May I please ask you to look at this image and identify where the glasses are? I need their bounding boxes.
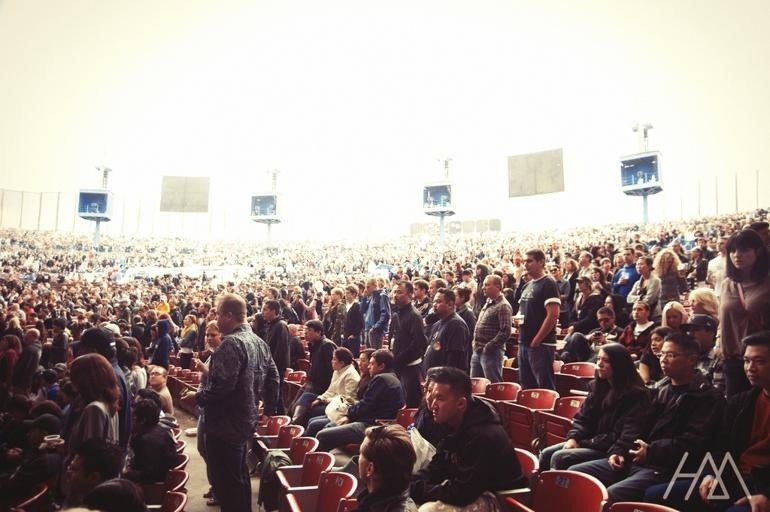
[655,350,696,360]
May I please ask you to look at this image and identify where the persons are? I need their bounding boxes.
[299,288,318,319]
[645,332,770,511]
[688,315,728,392]
[287,318,340,417]
[588,268,611,295]
[306,349,408,449]
[518,207,770,252]
[564,276,603,341]
[611,247,638,296]
[341,285,363,360]
[563,259,579,282]
[410,368,530,512]
[180,291,280,511]
[578,251,598,278]
[322,288,346,346]
[422,287,469,376]
[618,300,658,350]
[539,343,651,487]
[567,334,728,504]
[298,234,516,286]
[687,247,707,288]
[626,257,663,326]
[599,258,612,282]
[195,320,223,508]
[180,237,297,317]
[414,369,454,445]
[469,274,514,383]
[587,305,623,346]
[651,248,689,310]
[352,424,420,512]
[261,300,305,416]
[661,301,689,332]
[706,237,730,287]
[180,314,196,369]
[385,282,429,408]
[294,348,362,429]
[308,348,379,422]
[362,279,389,349]
[517,247,562,392]
[713,230,770,394]
[688,286,718,320]
[639,326,674,386]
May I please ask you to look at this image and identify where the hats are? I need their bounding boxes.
[678,314,718,332]
[66,327,115,348]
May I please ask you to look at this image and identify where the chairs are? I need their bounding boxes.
[474,382,521,412]
[496,470,609,512]
[554,362,596,396]
[257,425,304,461]
[499,388,561,450]
[258,400,263,407]
[10,482,49,512]
[147,492,187,512]
[501,365,520,383]
[375,408,420,429]
[258,407,264,416]
[253,416,291,438]
[283,471,358,512]
[266,436,320,464]
[276,452,335,489]
[535,395,588,447]
[168,470,189,491]
[470,377,491,395]
[513,447,540,486]
[175,440,186,455]
[173,452,190,470]
[282,367,307,407]
[167,364,200,392]
[609,501,679,512]
[174,428,182,439]
